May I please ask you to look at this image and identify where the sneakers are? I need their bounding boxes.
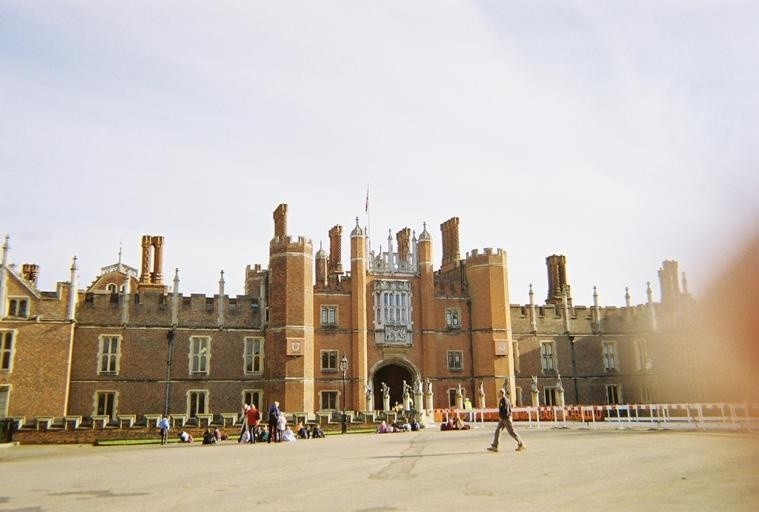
[515,444,525,451]
[487,447,498,452]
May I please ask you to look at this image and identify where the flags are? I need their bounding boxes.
[365,191,369,213]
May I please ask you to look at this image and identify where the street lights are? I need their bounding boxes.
[163,329,176,417]
[567,335,581,405]
[338,351,350,436]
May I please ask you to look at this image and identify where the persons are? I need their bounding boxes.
[244,403,260,445]
[341,411,347,434]
[176,426,229,444]
[487,388,526,452]
[463,397,473,409]
[158,413,171,445]
[374,412,465,434]
[236,402,251,444]
[268,400,281,443]
[276,410,287,442]
[253,419,325,442]
